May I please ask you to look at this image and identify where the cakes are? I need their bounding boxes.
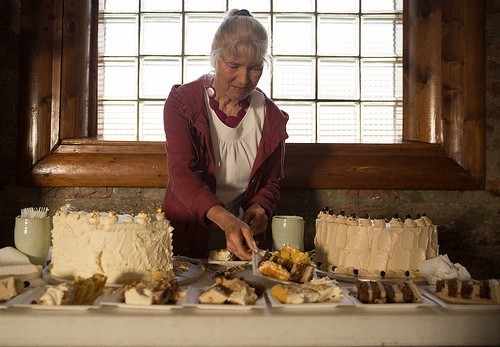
[270,275,342,304]
[357,281,422,303]
[433,278,500,303]
[39,273,108,305]
[313,207,439,279]
[51,203,173,285]
[121,278,179,306]
[199,277,257,306]
[258,245,313,283]
[0,274,25,300]
[208,248,239,261]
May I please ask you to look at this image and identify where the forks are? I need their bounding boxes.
[20,207,48,218]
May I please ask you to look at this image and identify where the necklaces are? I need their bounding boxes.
[206,85,249,128]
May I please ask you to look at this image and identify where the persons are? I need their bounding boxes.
[160,10,289,261]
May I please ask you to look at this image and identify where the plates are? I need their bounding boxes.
[207,255,253,268]
[182,286,268,311]
[420,284,500,310]
[100,281,185,310]
[344,286,437,309]
[9,284,104,312]
[266,286,355,309]
[251,248,316,285]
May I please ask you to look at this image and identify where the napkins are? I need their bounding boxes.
[0,247,38,276]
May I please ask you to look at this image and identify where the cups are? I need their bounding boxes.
[272,215,304,252]
[14,217,51,268]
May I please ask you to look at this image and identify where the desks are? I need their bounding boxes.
[0,258,500,347]
